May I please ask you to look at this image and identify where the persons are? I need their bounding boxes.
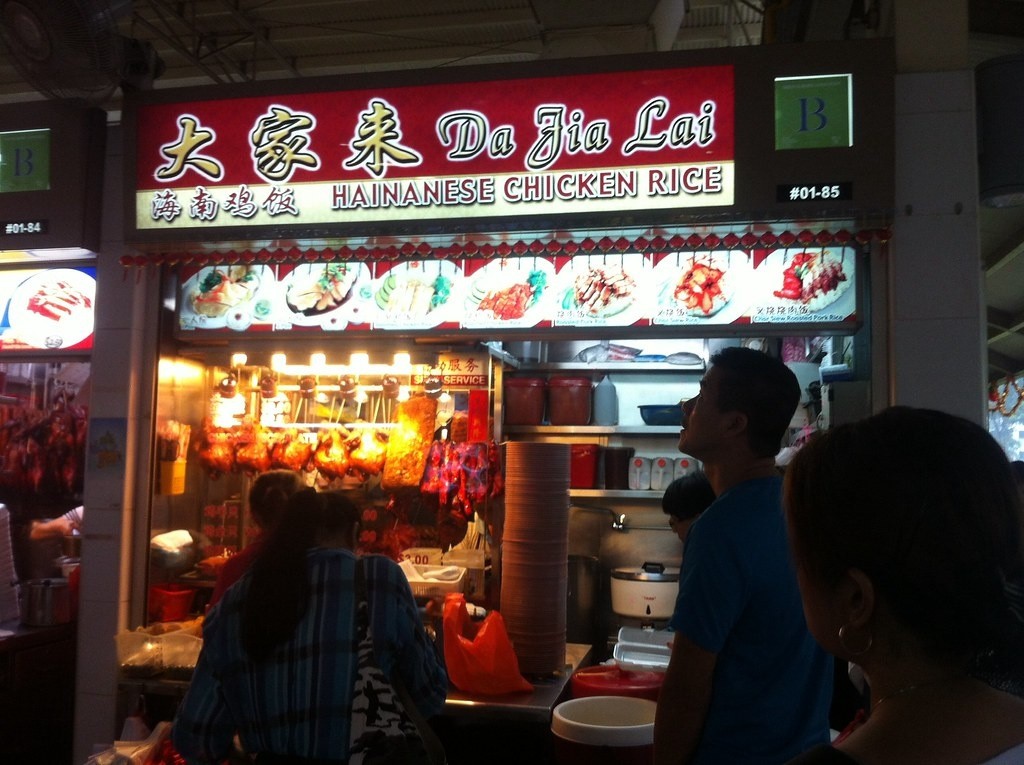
[653,348,1024,765]
[169,471,449,765]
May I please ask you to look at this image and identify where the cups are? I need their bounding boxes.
[161,461,186,495]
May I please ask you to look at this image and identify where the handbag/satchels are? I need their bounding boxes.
[425,592,534,696]
[347,555,447,765]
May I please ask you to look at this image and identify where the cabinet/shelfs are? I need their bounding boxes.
[524,357,705,501]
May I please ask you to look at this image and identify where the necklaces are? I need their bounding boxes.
[870,674,967,713]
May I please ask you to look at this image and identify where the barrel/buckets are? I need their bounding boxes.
[551,696,657,765]
[502,378,544,426]
[147,582,197,622]
[605,446,635,489]
[571,665,665,700]
[547,377,591,425]
[786,362,819,403]
[570,444,599,489]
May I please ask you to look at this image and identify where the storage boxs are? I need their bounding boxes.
[571,444,599,488]
[505,377,547,424]
[550,375,593,424]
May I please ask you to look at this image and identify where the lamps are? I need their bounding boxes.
[299,376,319,401]
[257,374,278,400]
[425,375,444,400]
[383,377,400,400]
[219,378,239,398]
[340,376,358,398]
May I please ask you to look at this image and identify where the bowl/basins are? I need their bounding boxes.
[638,405,685,424]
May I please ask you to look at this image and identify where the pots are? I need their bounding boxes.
[611,562,680,621]
[12,578,77,625]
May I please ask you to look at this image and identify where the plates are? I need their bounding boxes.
[556,254,650,326]
[8,268,96,349]
[278,263,371,326]
[652,250,751,324]
[465,257,556,328]
[181,265,275,329]
[753,248,855,322]
[375,260,462,330]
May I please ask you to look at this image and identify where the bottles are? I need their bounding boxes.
[629,457,651,490]
[650,458,674,490]
[674,458,698,480]
